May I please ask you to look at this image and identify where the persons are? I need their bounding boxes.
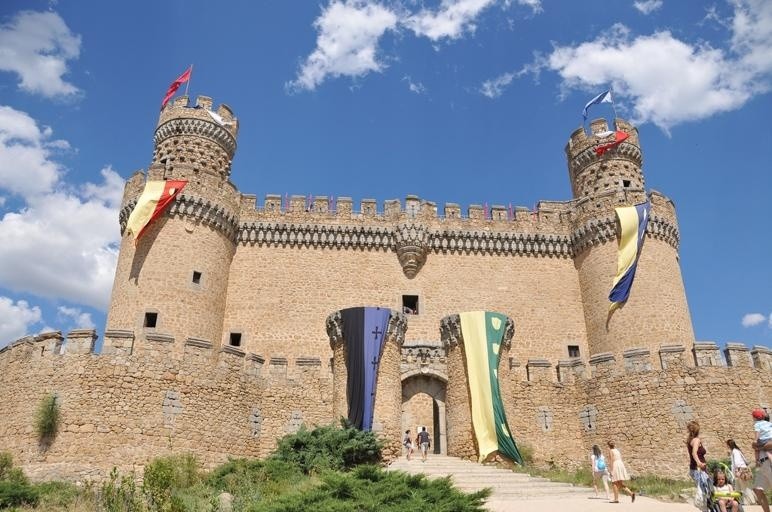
[686,420,712,512]
[418,426,431,461]
[590,444,611,500]
[750,409,772,512]
[607,440,635,504]
[752,410,772,467]
[727,439,752,505]
[711,468,739,512]
[403,430,414,461]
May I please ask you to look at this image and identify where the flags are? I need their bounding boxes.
[593,130,630,155]
[606,199,652,313]
[123,179,189,248]
[161,66,192,106]
[582,88,612,120]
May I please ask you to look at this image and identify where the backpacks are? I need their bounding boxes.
[597,457,605,470]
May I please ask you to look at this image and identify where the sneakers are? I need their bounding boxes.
[631,493,634,502]
[610,501,619,503]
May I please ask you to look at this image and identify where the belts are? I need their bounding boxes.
[759,457,768,463]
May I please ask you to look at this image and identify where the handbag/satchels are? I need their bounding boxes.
[735,467,752,480]
[694,488,707,510]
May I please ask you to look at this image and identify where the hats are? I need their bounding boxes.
[753,411,763,419]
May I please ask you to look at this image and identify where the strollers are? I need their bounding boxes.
[697,463,744,512]
